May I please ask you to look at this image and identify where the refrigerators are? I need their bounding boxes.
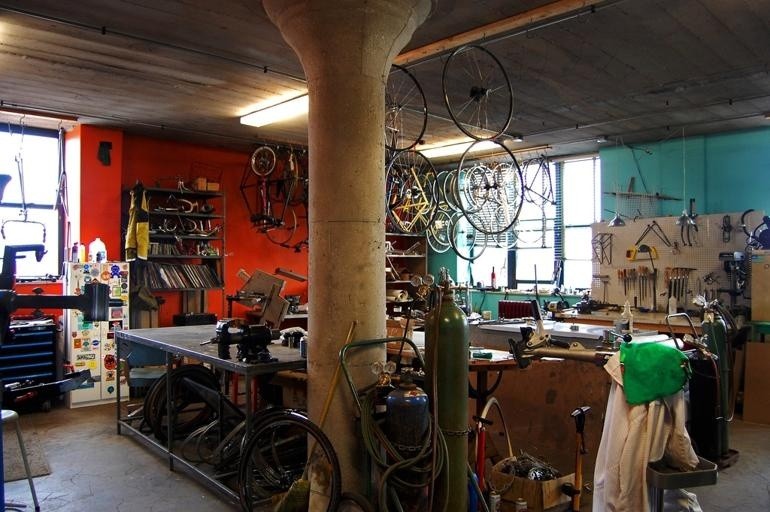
[62,261,132,408]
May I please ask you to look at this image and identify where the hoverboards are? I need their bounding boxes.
[248,145,289,234]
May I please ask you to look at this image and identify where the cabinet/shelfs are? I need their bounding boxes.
[123,188,226,293]
[385,233,428,303]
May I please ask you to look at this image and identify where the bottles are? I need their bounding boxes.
[668,296,677,315]
[515,498,528,512]
[491,267,496,290]
[621,301,633,333]
[300,336,308,358]
[489,492,502,512]
[72,238,106,263]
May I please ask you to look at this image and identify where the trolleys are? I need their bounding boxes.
[332,332,431,511]
[662,307,741,470]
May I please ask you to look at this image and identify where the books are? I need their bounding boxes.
[150,261,220,289]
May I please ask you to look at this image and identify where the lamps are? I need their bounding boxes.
[607,135,626,227]
[676,128,696,225]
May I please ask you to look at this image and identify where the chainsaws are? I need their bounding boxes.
[625,245,658,261]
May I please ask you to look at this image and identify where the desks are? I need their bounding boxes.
[554,306,732,336]
[115,324,308,512]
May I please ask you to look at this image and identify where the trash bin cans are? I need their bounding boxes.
[743,321,769,425]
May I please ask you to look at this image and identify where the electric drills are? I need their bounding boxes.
[199,318,281,363]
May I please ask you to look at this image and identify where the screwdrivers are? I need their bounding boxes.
[617,265,651,305]
